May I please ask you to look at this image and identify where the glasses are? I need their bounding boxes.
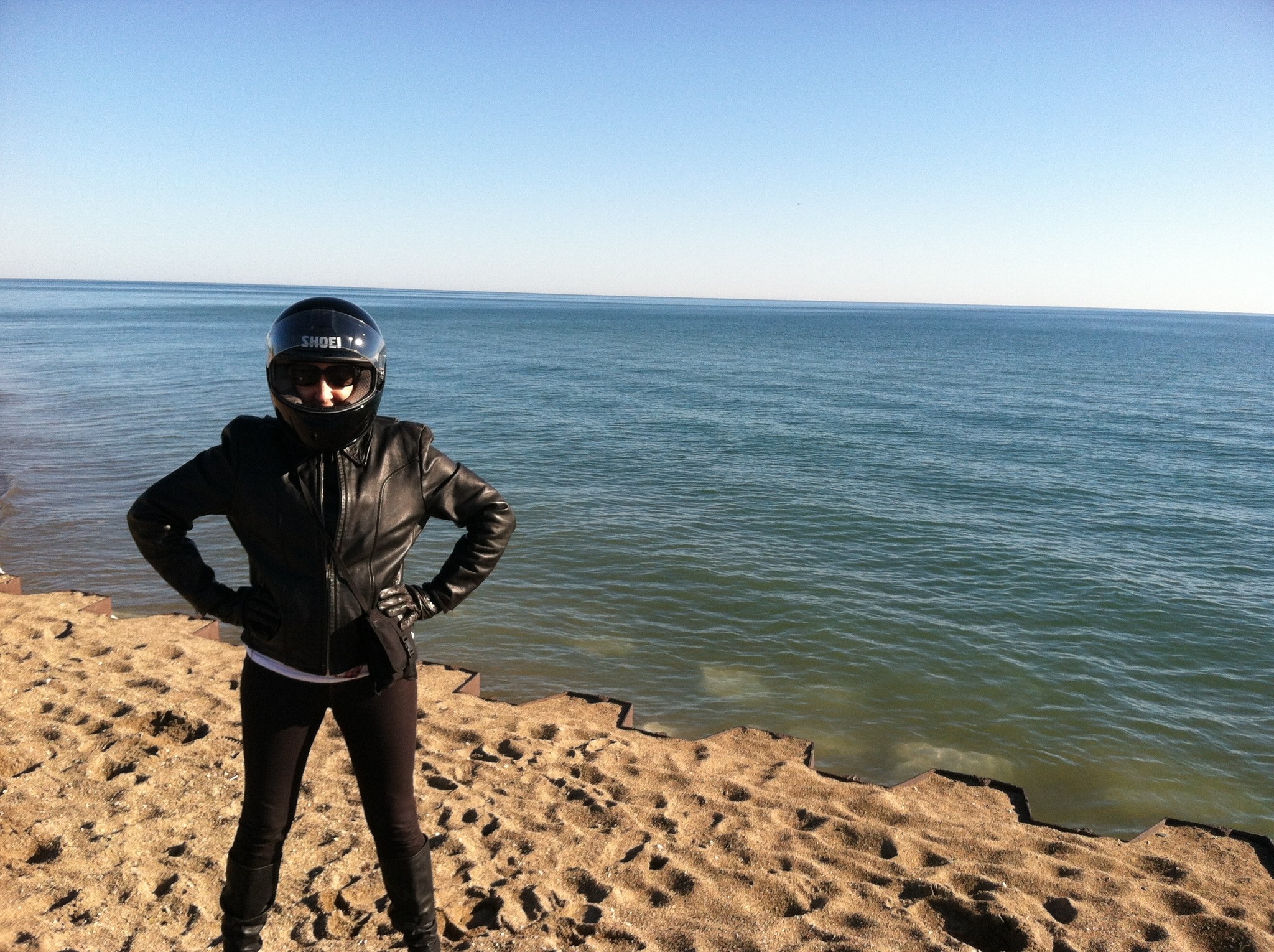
[285,364,363,388]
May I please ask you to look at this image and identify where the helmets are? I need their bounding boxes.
[265,297,386,448]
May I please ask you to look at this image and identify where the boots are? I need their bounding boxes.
[376,833,442,952]
[220,848,283,952]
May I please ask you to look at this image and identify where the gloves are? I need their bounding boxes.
[378,583,444,631]
[207,583,280,641]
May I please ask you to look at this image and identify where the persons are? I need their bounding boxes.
[126,295,518,952]
